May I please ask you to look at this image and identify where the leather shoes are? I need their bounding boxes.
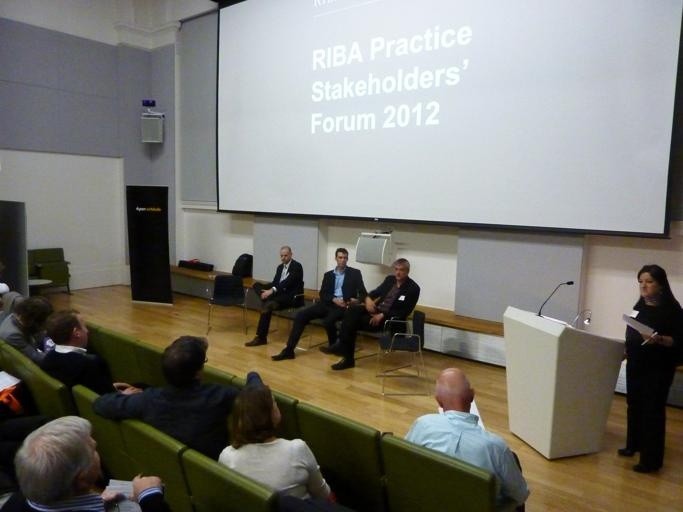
[319,344,344,356]
[245,336,267,346]
[618,447,641,457]
[633,463,658,472]
[271,350,294,360]
[331,358,354,370]
[258,301,279,315]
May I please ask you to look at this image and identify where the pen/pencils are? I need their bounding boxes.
[641,331,658,346]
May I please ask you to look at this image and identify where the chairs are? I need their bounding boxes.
[206,273,432,396]
[0,318,527,512]
[27,247,71,297]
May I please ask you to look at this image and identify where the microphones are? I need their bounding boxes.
[537,281,573,317]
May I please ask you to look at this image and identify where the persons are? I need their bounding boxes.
[269,248,367,360]
[1,297,54,365]
[402,367,530,511]
[90,334,264,464]
[318,258,420,371]
[615,264,683,474]
[0,414,173,512]
[39,308,157,397]
[243,245,303,347]
[216,381,336,506]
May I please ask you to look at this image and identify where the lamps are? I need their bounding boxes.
[570,308,593,328]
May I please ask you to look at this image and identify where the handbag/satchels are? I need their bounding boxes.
[232,254,252,279]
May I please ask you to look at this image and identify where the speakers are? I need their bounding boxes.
[141,117,163,143]
[354,232,392,267]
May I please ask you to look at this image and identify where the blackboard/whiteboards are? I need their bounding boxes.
[173,5,220,210]
